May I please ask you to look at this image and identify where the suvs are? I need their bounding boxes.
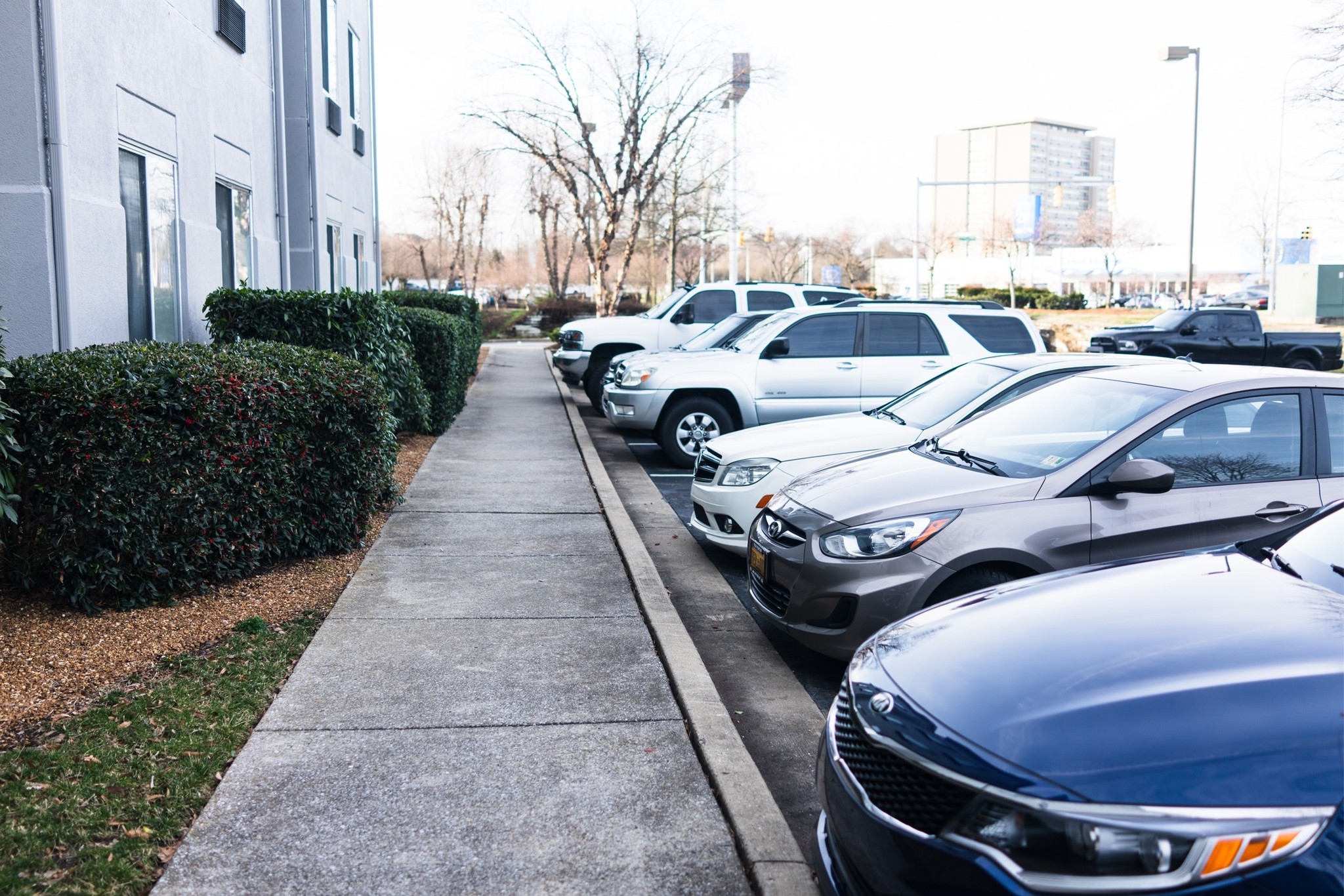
[554,280,869,417]
[600,296,1048,473]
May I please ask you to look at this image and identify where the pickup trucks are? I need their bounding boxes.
[1085,305,1343,374]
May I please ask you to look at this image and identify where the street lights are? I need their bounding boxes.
[1161,45,1201,308]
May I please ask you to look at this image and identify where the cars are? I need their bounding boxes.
[1101,284,1268,311]
[811,499,1344,896]
[688,351,1344,664]
